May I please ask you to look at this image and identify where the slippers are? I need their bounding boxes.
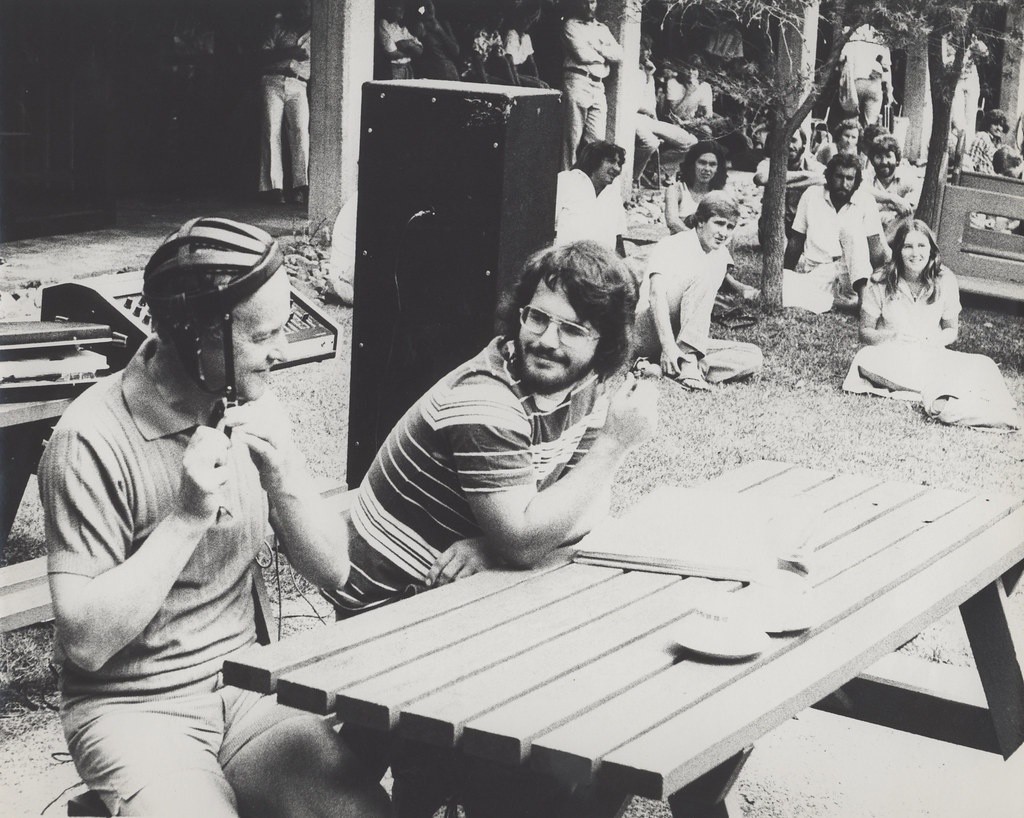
[742,286,761,299]
[674,376,712,393]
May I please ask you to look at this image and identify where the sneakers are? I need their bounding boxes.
[269,191,306,204]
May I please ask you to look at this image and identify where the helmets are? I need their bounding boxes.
[143,216,284,343]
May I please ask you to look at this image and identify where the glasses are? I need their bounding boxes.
[519,305,600,349]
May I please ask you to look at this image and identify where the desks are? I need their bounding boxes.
[219,459,1024,817]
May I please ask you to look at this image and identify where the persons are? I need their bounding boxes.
[318,239,660,818]
[842,219,1024,429]
[838,23,894,124]
[664,140,761,307]
[246,0,626,208]
[628,189,764,392]
[631,31,722,189]
[782,153,892,315]
[941,31,990,136]
[735,113,919,321]
[553,140,628,247]
[36,215,392,818]
[944,108,1024,251]
